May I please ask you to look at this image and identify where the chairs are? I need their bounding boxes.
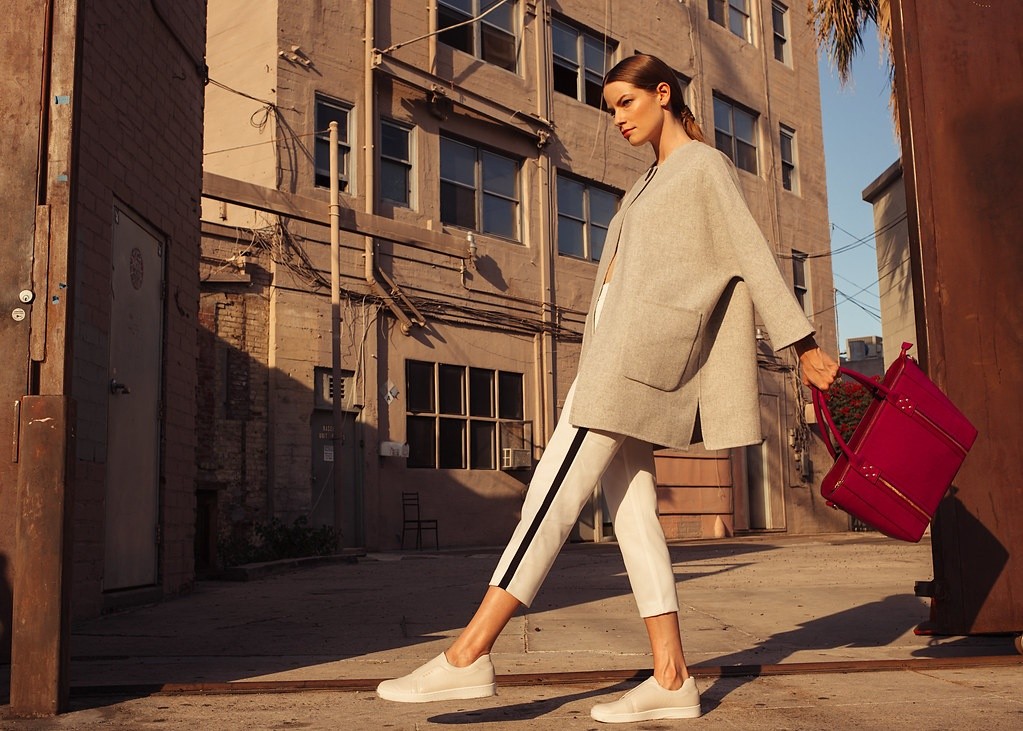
[399,489,439,551]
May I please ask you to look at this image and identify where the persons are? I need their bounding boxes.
[377,55,839,723]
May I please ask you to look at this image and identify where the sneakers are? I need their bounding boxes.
[590,675,702,724]
[376,651,498,703]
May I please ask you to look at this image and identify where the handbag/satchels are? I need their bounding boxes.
[812,342,979,543]
